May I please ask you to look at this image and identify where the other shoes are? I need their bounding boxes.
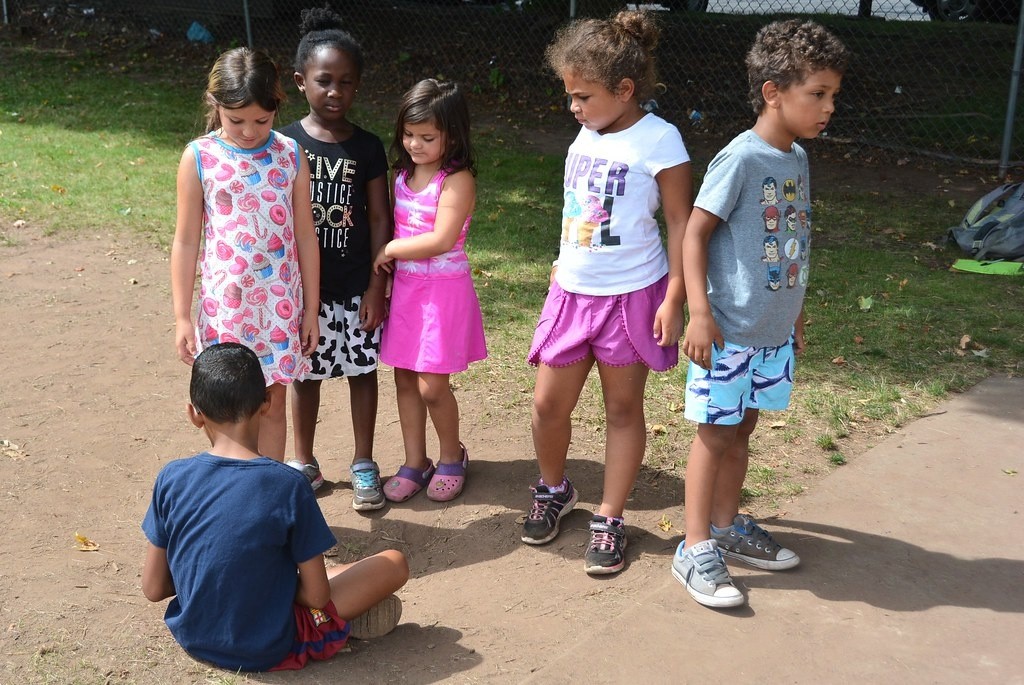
[348,593,403,640]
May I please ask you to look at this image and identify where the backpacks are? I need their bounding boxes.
[943,182,1023,267]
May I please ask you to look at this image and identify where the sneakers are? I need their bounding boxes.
[521,477,578,544]
[284,456,324,491]
[584,514,627,575]
[709,514,800,570]
[349,462,386,510]
[671,539,744,607]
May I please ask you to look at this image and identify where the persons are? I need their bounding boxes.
[520,12,692,575]
[171,47,320,464]
[276,6,389,511]
[373,77,488,502]
[671,16,848,608]
[142,342,409,673]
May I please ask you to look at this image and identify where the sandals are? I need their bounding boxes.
[383,458,436,502]
[427,441,469,501]
